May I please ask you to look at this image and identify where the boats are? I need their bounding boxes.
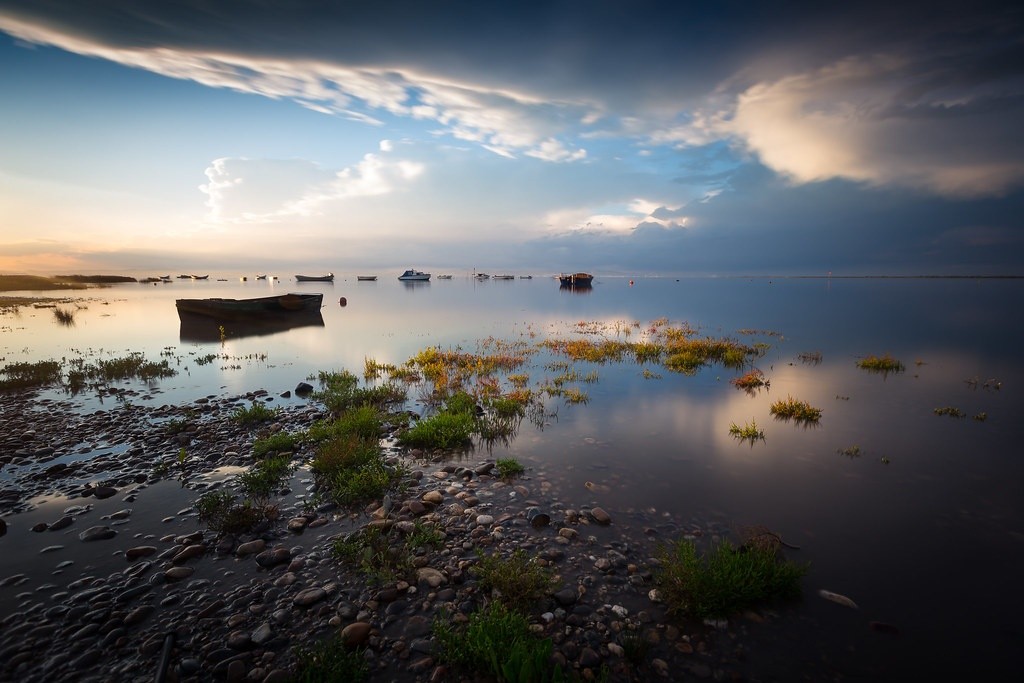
[160,275,170,279]
[437,274,453,279]
[295,272,335,282]
[397,268,431,280]
[192,274,209,279]
[492,273,514,279]
[175,291,323,323]
[257,274,267,279]
[177,275,192,278]
[357,275,378,281]
[217,279,228,282]
[474,273,491,280]
[163,279,173,283]
[559,272,594,287]
[520,275,533,280]
